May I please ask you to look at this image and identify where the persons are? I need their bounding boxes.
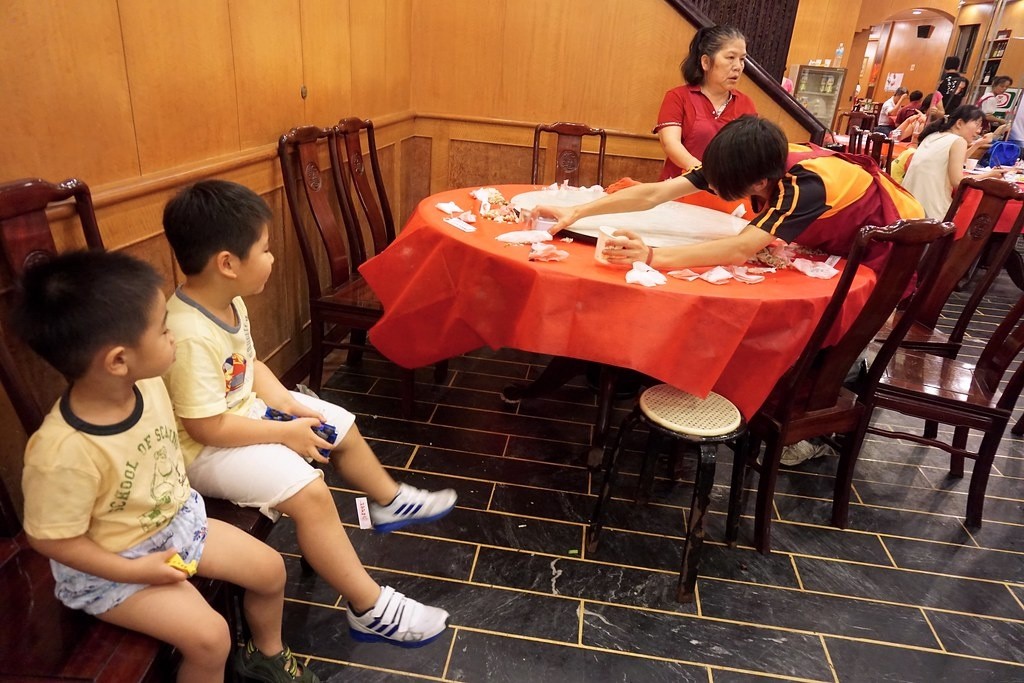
[651,23,758,201]
[878,56,1024,262]
[525,113,927,466]
[160,180,457,648]
[13,246,321,683]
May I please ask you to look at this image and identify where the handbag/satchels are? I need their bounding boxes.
[977,141,1020,167]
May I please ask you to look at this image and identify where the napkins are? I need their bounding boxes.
[791,258,839,279]
[731,203,747,219]
[667,268,700,282]
[626,260,667,288]
[733,267,765,285]
[496,229,552,243]
[434,201,464,215]
[460,210,476,223]
[529,242,569,263]
[700,265,732,285]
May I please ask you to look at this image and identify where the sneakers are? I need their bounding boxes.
[780,441,828,466]
[345,585,450,648]
[238,638,319,683]
[368,481,457,533]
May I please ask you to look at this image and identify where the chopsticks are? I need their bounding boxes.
[992,155,1006,180]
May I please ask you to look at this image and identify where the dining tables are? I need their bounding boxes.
[357,131,1024,500]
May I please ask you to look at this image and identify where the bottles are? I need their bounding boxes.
[993,43,1006,58]
[820,76,834,92]
[865,99,869,111]
[832,43,844,68]
[799,74,808,90]
[813,101,820,115]
[983,67,991,85]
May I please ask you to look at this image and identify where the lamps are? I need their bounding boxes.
[912,10,922,15]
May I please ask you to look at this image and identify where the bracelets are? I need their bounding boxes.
[646,246,653,265]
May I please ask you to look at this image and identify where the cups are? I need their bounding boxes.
[731,204,747,217]
[965,158,979,171]
[533,218,557,233]
[824,60,831,67]
[594,225,628,264]
[815,60,821,66]
[893,130,902,143]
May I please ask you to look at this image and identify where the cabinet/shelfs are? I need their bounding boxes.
[793,65,848,131]
[968,37,1024,106]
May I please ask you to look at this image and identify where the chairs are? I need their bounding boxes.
[872,176,1024,440]
[840,293,1024,529]
[532,121,606,188]
[736,219,957,552]
[864,132,895,177]
[848,125,864,154]
[279,124,448,413]
[0,179,279,683]
[334,116,395,363]
[873,103,884,127]
[836,111,875,136]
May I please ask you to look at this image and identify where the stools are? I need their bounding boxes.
[588,384,746,602]
